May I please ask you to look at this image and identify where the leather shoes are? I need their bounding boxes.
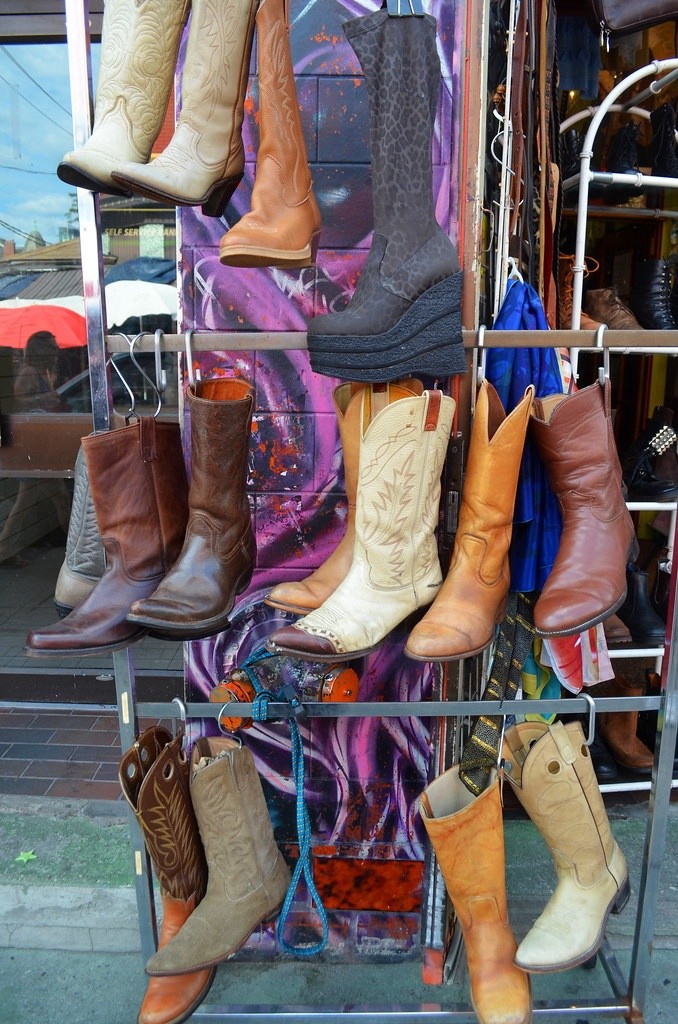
[584,287,646,330]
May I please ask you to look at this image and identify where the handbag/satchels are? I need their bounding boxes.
[581,0,677,56]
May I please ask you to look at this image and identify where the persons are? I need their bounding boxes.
[0,331,72,562]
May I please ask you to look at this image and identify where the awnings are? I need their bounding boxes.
[17,270,84,299]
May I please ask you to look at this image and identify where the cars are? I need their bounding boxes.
[53,349,178,415]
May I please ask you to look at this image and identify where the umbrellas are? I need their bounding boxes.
[0,296,86,329]
[0,305,87,349]
[104,280,178,399]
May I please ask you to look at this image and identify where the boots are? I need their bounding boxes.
[602,118,647,205]
[649,101,678,178]
[418,761,534,1024]
[263,379,424,618]
[556,251,607,330]
[528,377,641,639]
[53,442,112,622]
[143,736,292,976]
[218,0,323,270]
[126,377,258,640]
[403,377,537,663]
[22,414,192,659]
[306,6,469,385]
[565,129,598,178]
[625,418,676,507]
[498,719,631,975]
[629,255,678,330]
[567,557,671,781]
[110,0,260,218]
[651,405,677,486]
[263,381,460,665]
[56,0,191,200]
[116,724,217,1024]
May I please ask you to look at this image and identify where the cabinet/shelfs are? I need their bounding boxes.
[558,54,678,794]
